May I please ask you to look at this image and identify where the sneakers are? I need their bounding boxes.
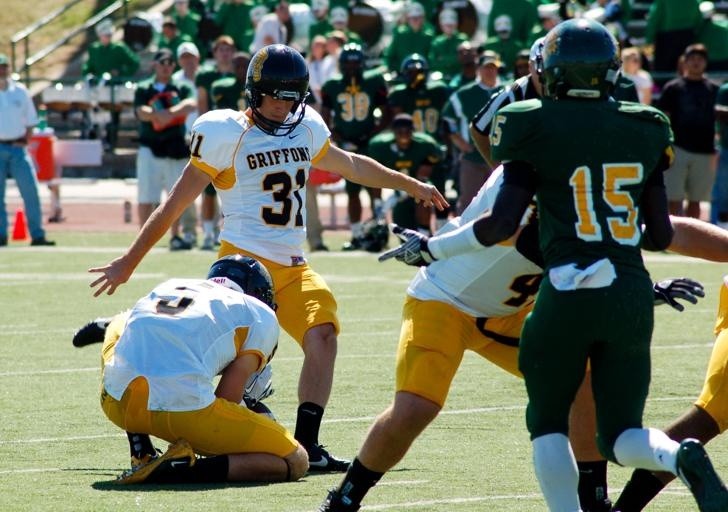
[590,499,614,512]
[322,488,361,512]
[307,443,351,473]
[73,319,108,347]
[183,233,196,244]
[676,439,728,511]
[342,240,360,251]
[214,235,220,244]
[171,237,191,250]
[245,393,276,419]
[118,438,196,483]
[201,238,214,251]
[131,449,160,469]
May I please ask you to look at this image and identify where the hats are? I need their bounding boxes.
[529,38,544,60]
[479,49,499,67]
[685,44,707,56]
[406,2,424,16]
[178,41,200,59]
[392,113,414,129]
[1,54,8,66]
[494,14,512,32]
[439,10,458,25]
[154,48,175,62]
[330,8,349,23]
[95,18,115,35]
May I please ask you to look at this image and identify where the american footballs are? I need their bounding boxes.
[254,401,276,419]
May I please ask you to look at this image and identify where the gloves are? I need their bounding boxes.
[378,222,439,268]
[654,276,706,312]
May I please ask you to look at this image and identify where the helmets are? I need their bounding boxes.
[538,17,620,101]
[207,253,278,311]
[400,53,429,88]
[245,45,310,136]
[338,43,365,78]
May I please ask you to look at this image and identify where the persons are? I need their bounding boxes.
[72,45,451,475]
[378,19,728,512]
[319,161,728,512]
[468,39,542,172]
[83,19,142,162]
[613,49,652,108]
[133,46,198,252]
[345,0,630,84]
[93,253,308,489]
[369,114,443,241]
[439,52,507,218]
[127,1,318,71]
[195,34,233,250]
[213,53,253,114]
[612,273,728,512]
[710,81,728,226]
[320,44,390,253]
[0,53,57,245]
[306,1,349,108]
[655,44,716,219]
[386,53,453,230]
[179,41,221,248]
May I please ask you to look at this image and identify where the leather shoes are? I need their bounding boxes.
[0,237,8,247]
[317,244,327,252]
[32,237,56,245]
